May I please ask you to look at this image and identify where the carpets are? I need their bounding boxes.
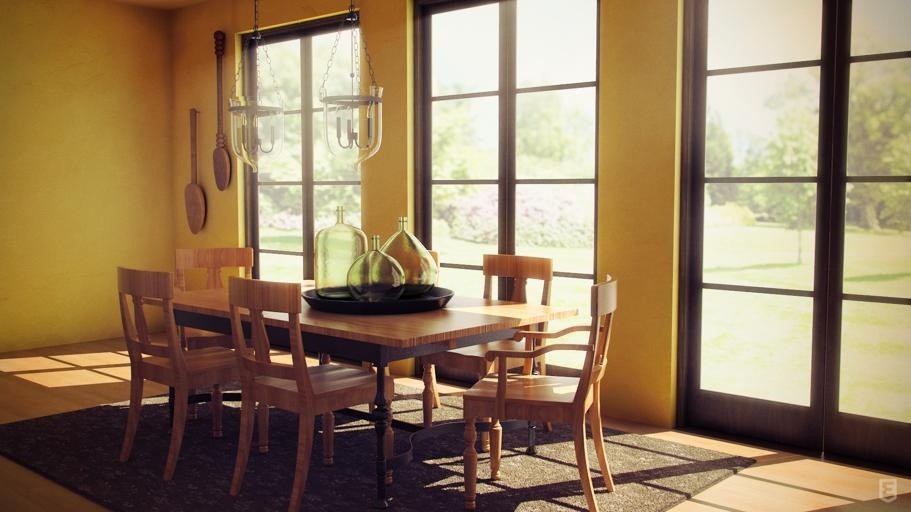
[0,382,756,512]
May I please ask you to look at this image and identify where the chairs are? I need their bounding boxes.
[462,274,618,511]
[173,247,255,424]
[374,252,441,408]
[115,266,254,482]
[421,253,554,451]
[227,274,395,511]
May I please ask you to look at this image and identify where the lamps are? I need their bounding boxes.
[227,1,385,174]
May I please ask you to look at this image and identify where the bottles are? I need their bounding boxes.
[312,205,368,297]
[348,234,404,300]
[372,215,437,295]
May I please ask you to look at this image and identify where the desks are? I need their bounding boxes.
[171,279,580,511]
